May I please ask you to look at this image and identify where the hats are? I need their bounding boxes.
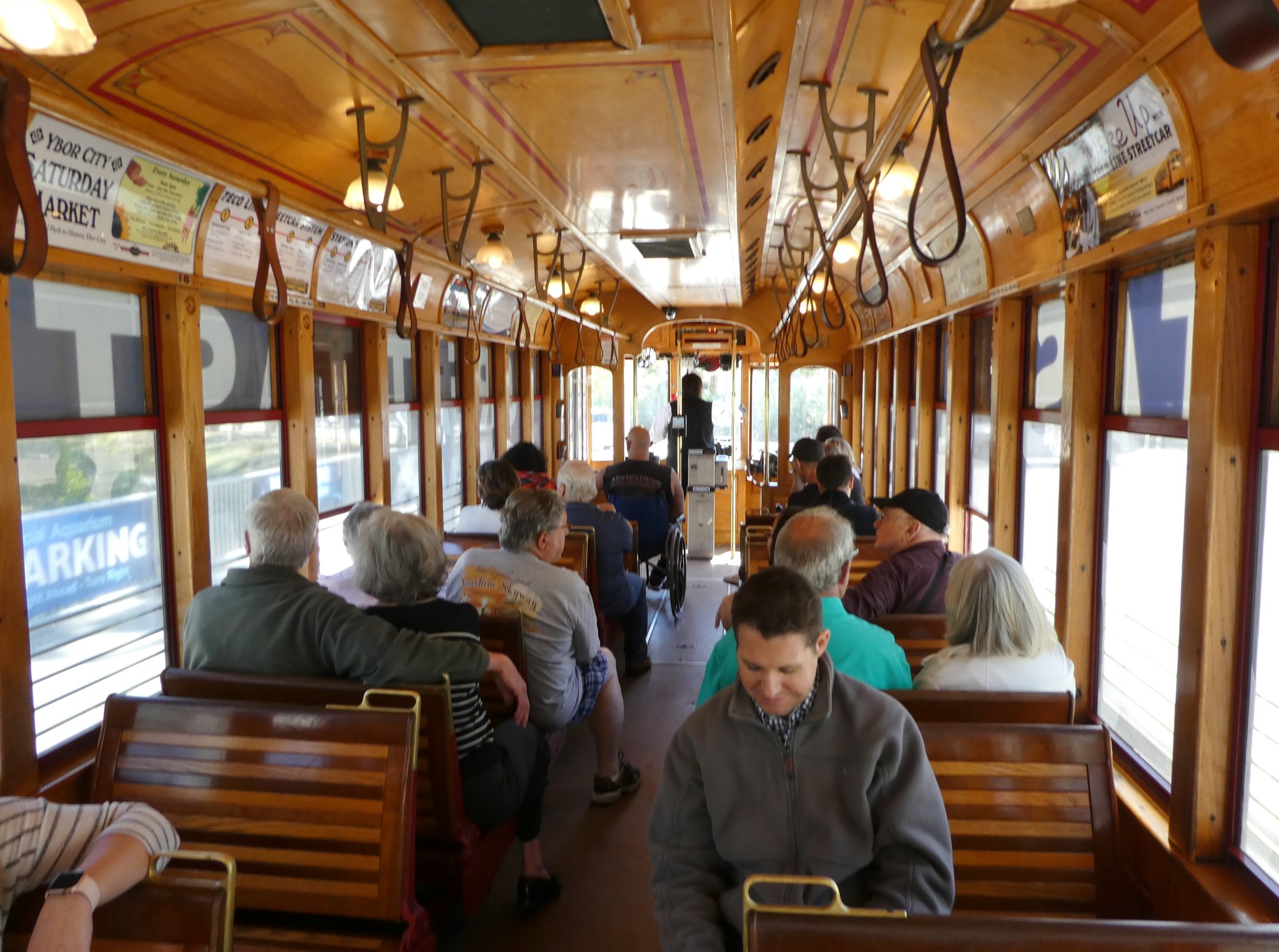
[788,437,824,462]
[870,488,950,537]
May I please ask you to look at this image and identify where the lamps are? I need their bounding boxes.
[833,231,862,263]
[800,295,818,314]
[542,263,572,299]
[579,292,604,315]
[867,129,926,203]
[809,268,834,294]
[0,0,97,58]
[342,147,403,213]
[475,224,516,269]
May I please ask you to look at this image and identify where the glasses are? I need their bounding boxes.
[875,510,887,521]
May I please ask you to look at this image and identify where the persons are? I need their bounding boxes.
[183,489,531,732]
[351,511,563,918]
[647,373,724,506]
[722,425,878,587]
[556,459,652,678]
[0,794,181,952]
[915,546,1076,694]
[499,441,557,491]
[648,566,954,952]
[318,500,385,609]
[455,460,521,534]
[438,487,642,805]
[595,426,685,590]
[696,506,914,708]
[840,488,964,620]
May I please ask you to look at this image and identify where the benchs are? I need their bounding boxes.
[0,480,1279,952]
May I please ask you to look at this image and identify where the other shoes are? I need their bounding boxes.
[516,871,560,914]
[647,568,667,590]
[625,657,654,678]
[723,573,741,586]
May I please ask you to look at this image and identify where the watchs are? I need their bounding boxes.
[45,870,101,913]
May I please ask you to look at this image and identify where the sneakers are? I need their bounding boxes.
[589,751,642,805]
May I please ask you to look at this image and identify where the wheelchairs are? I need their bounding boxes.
[638,514,690,616]
